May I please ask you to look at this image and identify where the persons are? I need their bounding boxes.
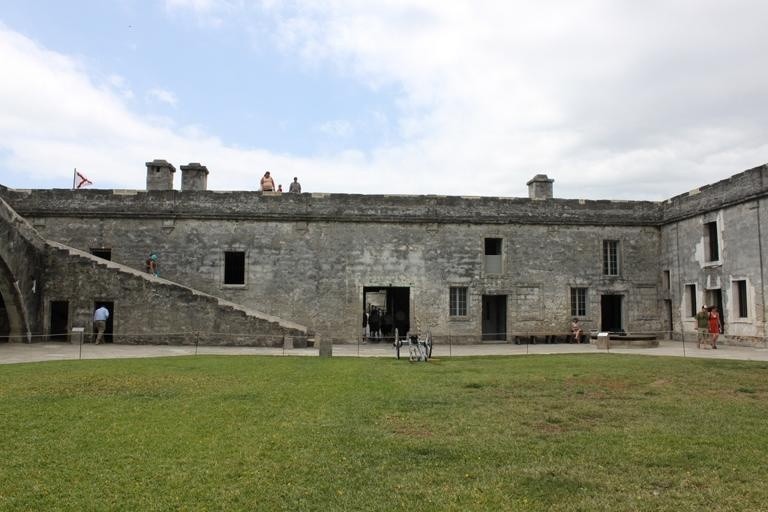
[92,305,109,344]
[572,318,582,343]
[288,176,301,193]
[707,305,722,349]
[694,305,709,350]
[362,306,407,344]
[144,254,154,274]
[260,171,274,192]
[276,184,282,192]
[150,255,160,276]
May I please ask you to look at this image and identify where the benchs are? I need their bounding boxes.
[512,331,590,344]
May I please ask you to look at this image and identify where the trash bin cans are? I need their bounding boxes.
[71,327,84,345]
[597,332,609,349]
